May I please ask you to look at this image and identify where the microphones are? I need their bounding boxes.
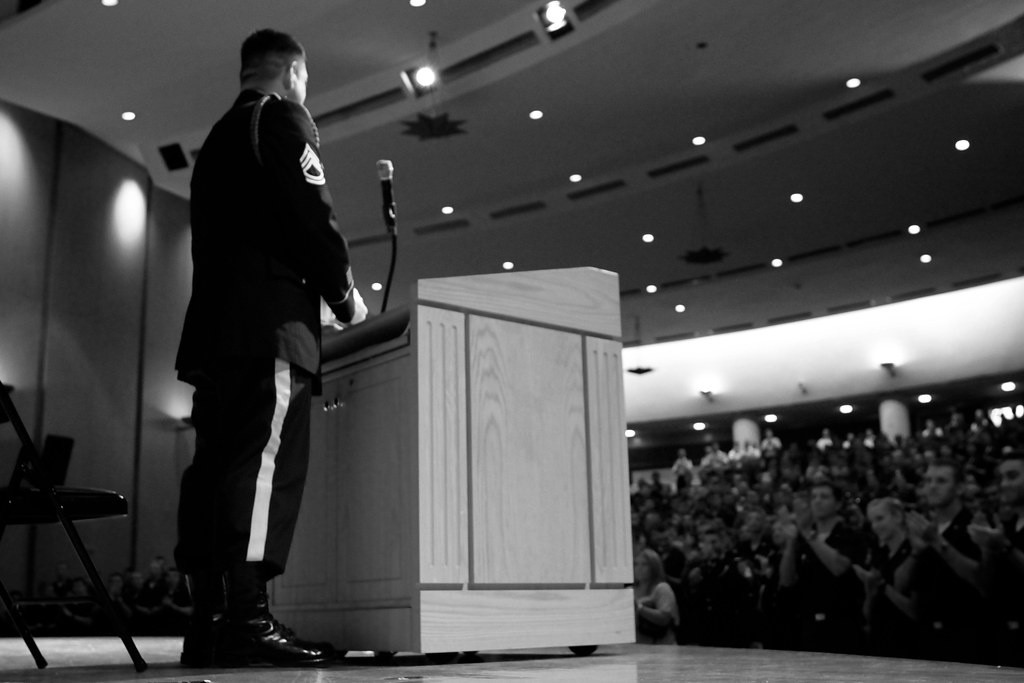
[376,160,397,234]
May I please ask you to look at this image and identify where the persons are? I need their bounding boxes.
[0,562,194,638]
[174,27,368,666]
[630,410,1024,665]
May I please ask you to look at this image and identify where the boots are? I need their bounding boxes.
[179,569,230,668]
[223,569,336,667]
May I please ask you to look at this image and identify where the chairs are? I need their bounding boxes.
[0,381,150,673]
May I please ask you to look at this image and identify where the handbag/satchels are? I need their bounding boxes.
[637,583,671,641]
